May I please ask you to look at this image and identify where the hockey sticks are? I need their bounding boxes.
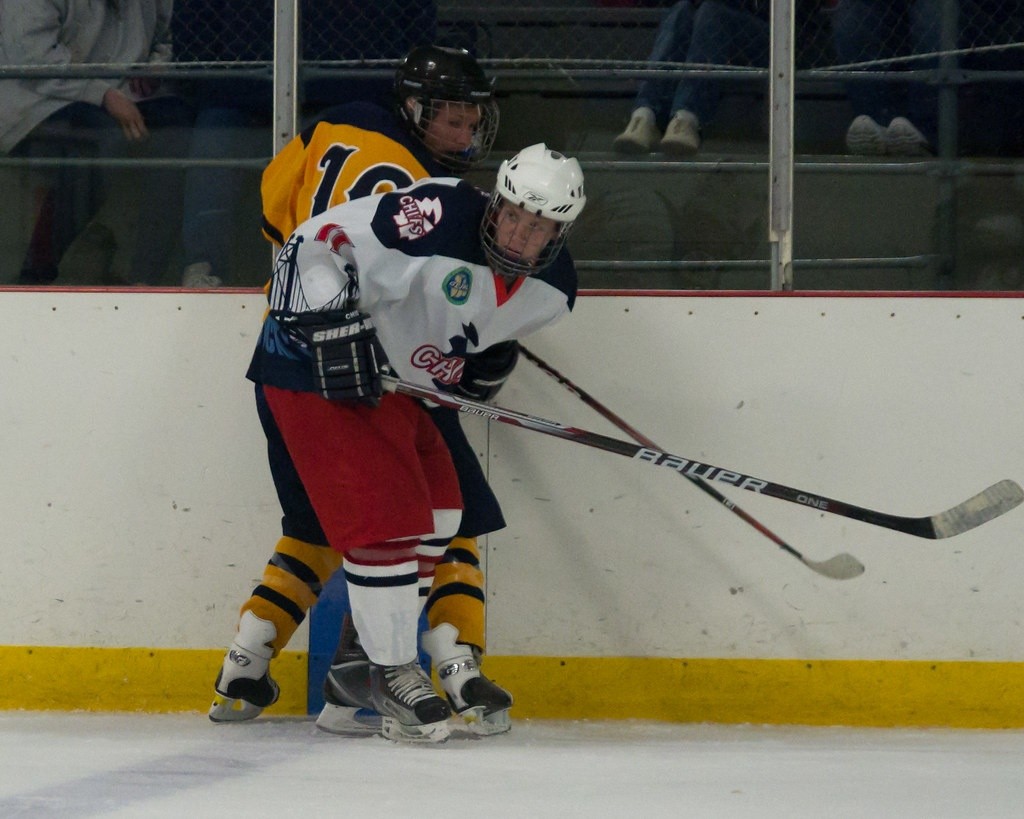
[380,374,1024,539]
[517,346,866,582]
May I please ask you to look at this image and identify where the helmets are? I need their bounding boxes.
[393,41,494,144]
[496,143,587,221]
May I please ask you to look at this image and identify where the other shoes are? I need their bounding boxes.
[846,114,887,156]
[886,116,929,156]
[182,260,222,290]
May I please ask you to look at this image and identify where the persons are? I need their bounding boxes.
[846,5,936,157]
[611,2,745,153]
[1,0,225,287]
[246,143,589,745]
[208,42,512,737]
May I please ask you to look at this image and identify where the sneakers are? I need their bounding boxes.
[370,658,455,742]
[662,118,702,155]
[613,107,656,155]
[209,609,280,722]
[422,622,512,735]
[316,611,388,736]
